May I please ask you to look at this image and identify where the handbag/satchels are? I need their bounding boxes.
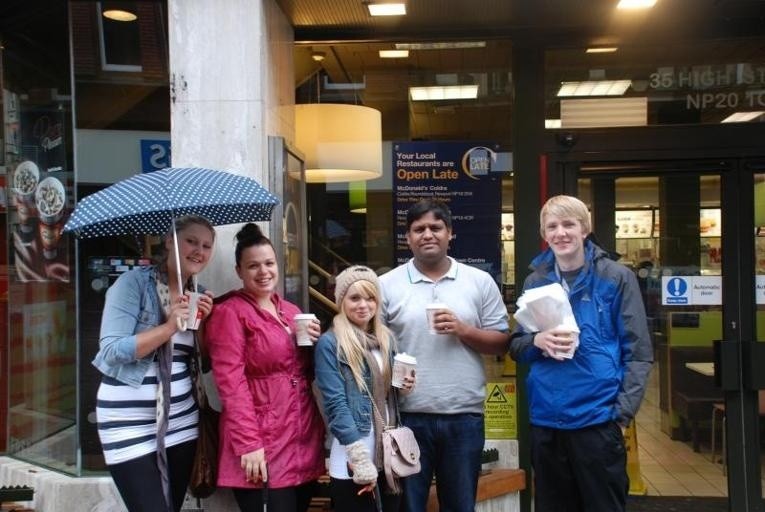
[381,423,424,481]
[187,370,220,501]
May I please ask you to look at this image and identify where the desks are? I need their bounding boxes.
[686,361,715,376]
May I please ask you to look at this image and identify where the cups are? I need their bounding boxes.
[13,160,66,261]
[390,350,416,389]
[425,299,447,335]
[555,323,580,358]
[293,312,317,346]
[184,290,207,331]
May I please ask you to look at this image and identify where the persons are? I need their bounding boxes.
[89,212,218,512]
[376,198,511,511]
[313,264,418,511]
[201,221,322,511]
[506,193,657,511]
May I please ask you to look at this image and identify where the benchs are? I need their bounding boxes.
[671,346,726,453]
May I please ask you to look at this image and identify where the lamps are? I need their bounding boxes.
[269,105,296,181]
[296,52,384,183]
[102,0,138,22]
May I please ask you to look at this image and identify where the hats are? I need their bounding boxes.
[333,263,382,311]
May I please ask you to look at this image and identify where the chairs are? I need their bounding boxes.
[711,389,765,476]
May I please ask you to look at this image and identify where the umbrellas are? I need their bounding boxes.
[57,166,283,334]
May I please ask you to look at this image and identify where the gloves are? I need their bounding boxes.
[345,437,381,488]
